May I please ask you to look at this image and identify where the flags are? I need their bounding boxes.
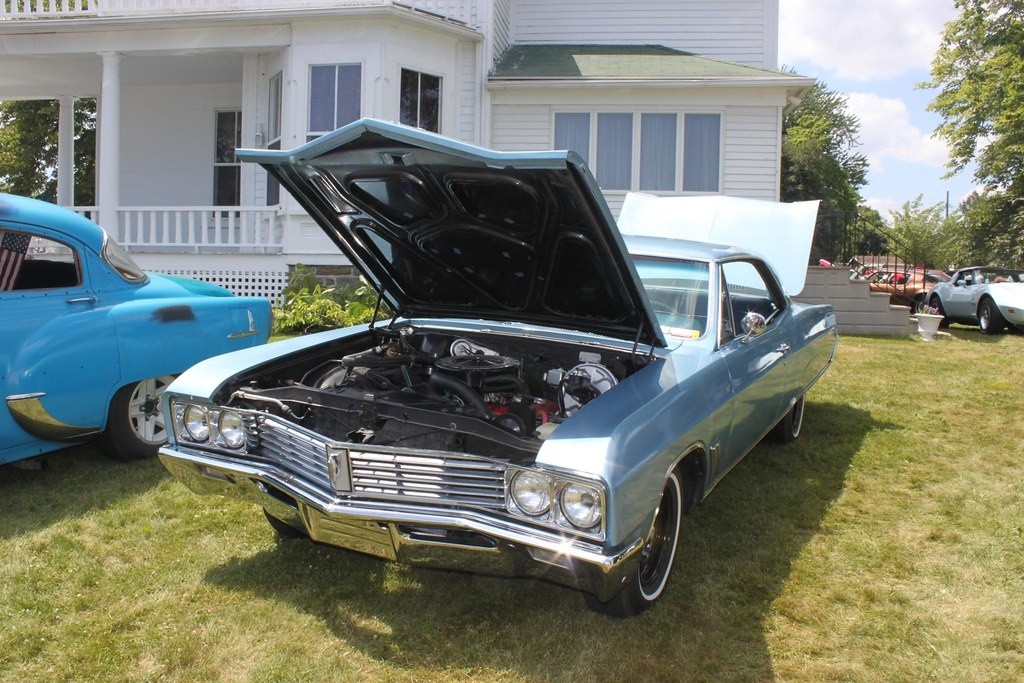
[0,229,34,293]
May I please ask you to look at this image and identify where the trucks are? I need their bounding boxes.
[850,264,886,281]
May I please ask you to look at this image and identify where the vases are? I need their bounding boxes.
[913,313,945,342]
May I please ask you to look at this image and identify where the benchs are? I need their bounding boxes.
[648,288,771,334]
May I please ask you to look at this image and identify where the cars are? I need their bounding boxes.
[0,193,274,468]
[926,267,1024,334]
[864,269,946,316]
[156,119,837,619]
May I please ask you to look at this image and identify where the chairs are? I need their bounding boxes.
[15,259,79,288]
[965,280,972,284]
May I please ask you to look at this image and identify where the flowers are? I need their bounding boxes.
[918,303,941,315]
